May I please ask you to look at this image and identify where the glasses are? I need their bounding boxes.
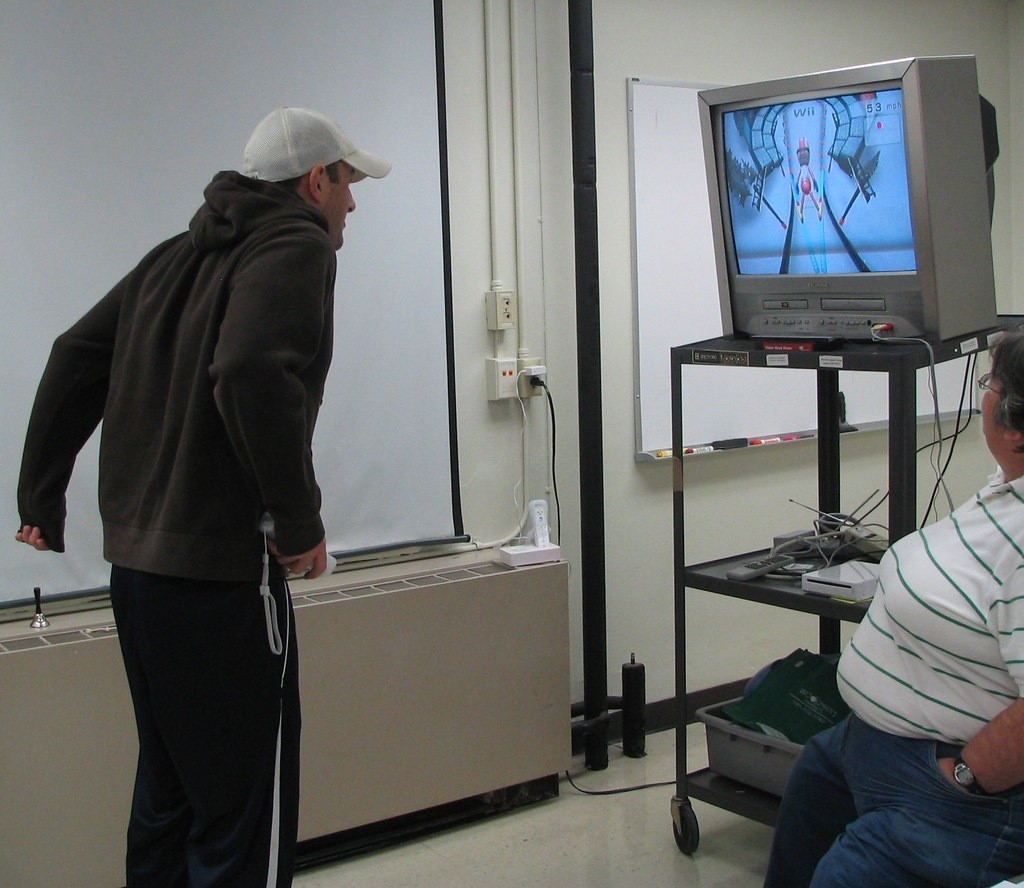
[977,372,1001,396]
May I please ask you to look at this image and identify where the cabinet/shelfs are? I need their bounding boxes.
[669,312,1023,857]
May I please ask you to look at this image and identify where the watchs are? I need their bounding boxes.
[952,754,988,796]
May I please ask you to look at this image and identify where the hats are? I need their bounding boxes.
[241,105,392,183]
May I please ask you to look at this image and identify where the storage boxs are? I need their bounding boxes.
[694,695,804,797]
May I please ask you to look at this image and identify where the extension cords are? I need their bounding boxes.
[844,525,888,560]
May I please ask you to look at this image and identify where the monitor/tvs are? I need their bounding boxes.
[696,53,998,343]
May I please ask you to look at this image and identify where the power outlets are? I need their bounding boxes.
[485,288,516,331]
[517,357,542,398]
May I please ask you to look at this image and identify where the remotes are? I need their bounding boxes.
[727,556,793,582]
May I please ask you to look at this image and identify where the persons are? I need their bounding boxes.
[762,326,1024,888]
[14,104,398,888]
